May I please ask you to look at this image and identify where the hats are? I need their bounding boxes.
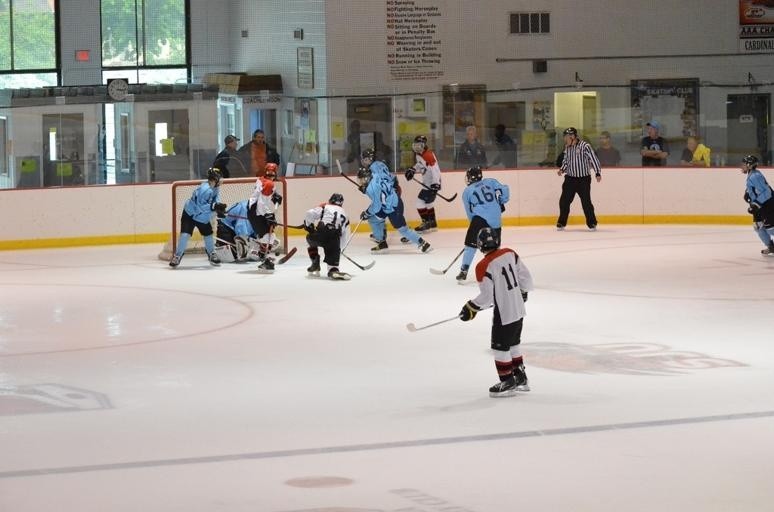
[225,135,240,144]
[646,121,659,129]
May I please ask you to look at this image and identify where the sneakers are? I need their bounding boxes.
[761,244,774,254]
[513,370,527,385]
[456,273,467,280]
[489,376,516,393]
[170,221,437,280]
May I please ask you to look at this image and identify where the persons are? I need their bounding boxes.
[459,225,534,393]
[639,121,670,167]
[541,131,623,168]
[357,166,431,253]
[215,192,282,262]
[681,136,712,168]
[455,165,509,281]
[357,147,412,243]
[169,167,229,267]
[208,129,281,179]
[405,133,442,231]
[739,153,774,256]
[246,162,282,270]
[553,126,604,230]
[303,191,353,279]
[453,124,488,170]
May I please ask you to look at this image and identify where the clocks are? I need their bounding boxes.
[107,78,128,101]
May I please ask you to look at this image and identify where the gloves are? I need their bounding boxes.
[748,201,760,214]
[743,193,751,202]
[217,211,228,218]
[405,167,416,181]
[265,213,277,227]
[359,184,367,193]
[460,300,481,321]
[430,184,440,195]
[272,194,282,205]
[359,210,371,220]
[212,202,227,211]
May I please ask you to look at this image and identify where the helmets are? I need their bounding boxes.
[477,227,499,250]
[744,155,759,166]
[362,148,375,166]
[357,166,372,185]
[412,135,427,153]
[329,193,343,205]
[208,167,224,183]
[265,163,278,175]
[563,127,577,134]
[464,165,482,183]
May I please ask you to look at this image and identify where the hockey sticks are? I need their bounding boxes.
[406,305,496,333]
[211,236,298,264]
[429,248,465,274]
[411,177,457,202]
[337,158,361,187]
[341,252,375,271]
[223,214,304,229]
